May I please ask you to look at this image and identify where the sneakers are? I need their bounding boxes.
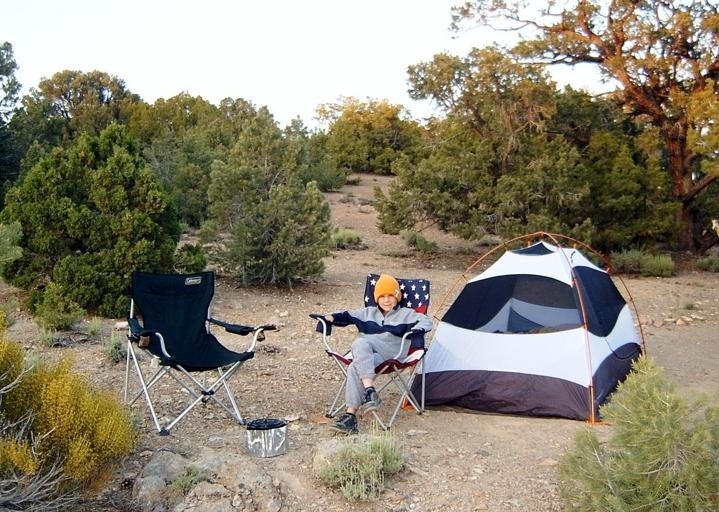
[328,412,358,434]
[362,390,382,414]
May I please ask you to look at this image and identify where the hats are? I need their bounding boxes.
[374,272,403,304]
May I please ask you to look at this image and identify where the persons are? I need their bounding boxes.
[324,275,432,434]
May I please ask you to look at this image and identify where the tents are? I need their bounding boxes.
[402,229,644,425]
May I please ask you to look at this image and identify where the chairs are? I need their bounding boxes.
[309,272,430,433]
[123,269,276,435]
